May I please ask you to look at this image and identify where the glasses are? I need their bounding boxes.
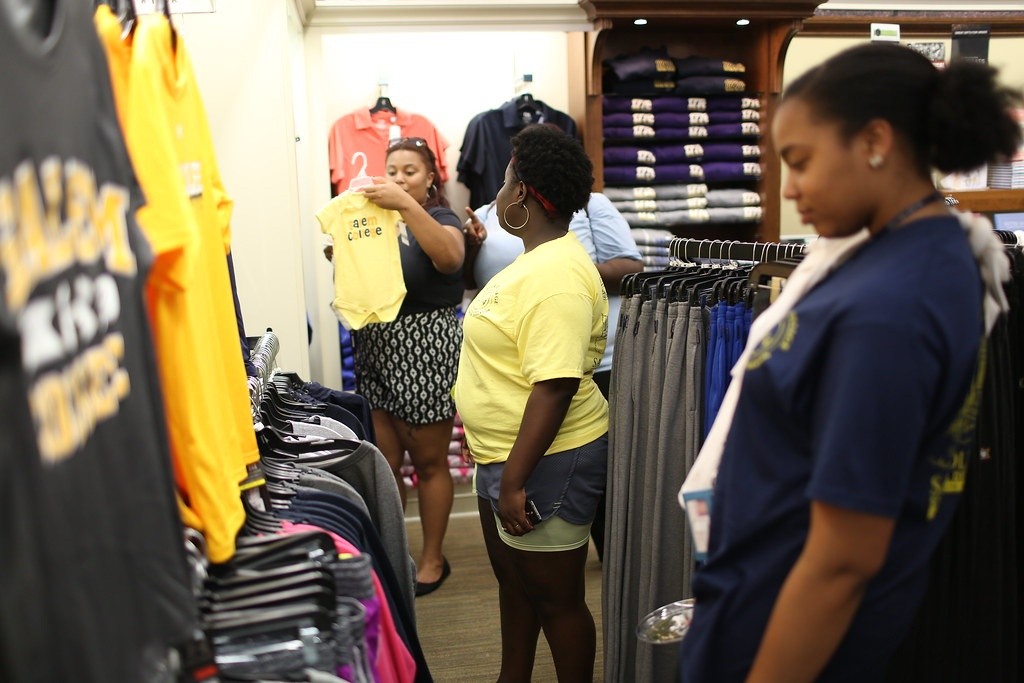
[388,137,428,149]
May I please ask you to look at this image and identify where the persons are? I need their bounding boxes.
[675,39,1024,683]
[462,195,643,564]
[451,124,611,683]
[324,138,474,595]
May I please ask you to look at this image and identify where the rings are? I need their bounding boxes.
[502,527,508,530]
[514,524,519,528]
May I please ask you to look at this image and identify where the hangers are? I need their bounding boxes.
[621,236,805,304]
[344,152,377,190]
[515,80,542,113]
[183,329,340,678]
[110,0,168,38]
[369,85,397,115]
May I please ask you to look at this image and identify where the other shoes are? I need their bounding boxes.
[416,555,451,596]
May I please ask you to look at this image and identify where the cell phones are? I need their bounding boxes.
[523,500,542,526]
